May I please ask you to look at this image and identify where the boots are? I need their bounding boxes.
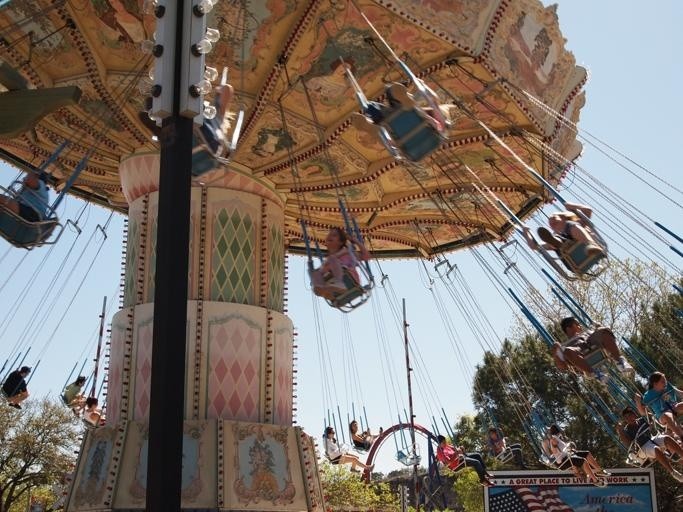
[570,224,603,256]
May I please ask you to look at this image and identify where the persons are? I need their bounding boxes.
[0,168,47,222]
[139,82,234,158]
[304,224,370,303]
[346,78,450,151]
[632,369,683,443]
[543,314,636,382]
[322,426,375,473]
[540,424,611,490]
[350,419,382,450]
[436,435,496,488]
[0,365,29,410]
[63,376,87,418]
[485,428,529,470]
[518,199,606,274]
[618,406,683,485]
[82,396,105,427]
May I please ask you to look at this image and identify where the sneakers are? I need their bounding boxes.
[596,469,612,477]
[389,83,417,112]
[350,467,361,473]
[483,473,495,479]
[592,369,609,384]
[310,283,336,300]
[615,356,633,373]
[514,462,531,470]
[367,464,375,472]
[670,469,683,482]
[592,479,609,488]
[478,479,496,488]
[537,226,564,248]
[323,276,347,294]
[9,403,22,410]
